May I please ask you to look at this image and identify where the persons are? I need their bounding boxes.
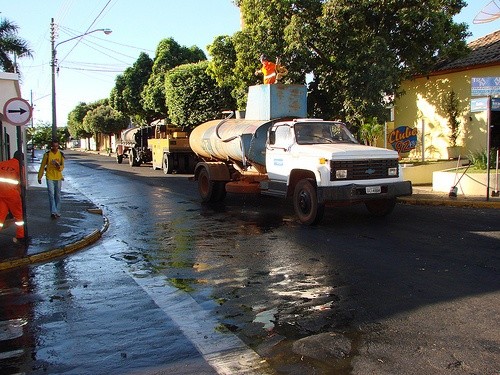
[37,138,65,219]
[318,128,340,142]
[255,55,280,84]
[0,151,31,242]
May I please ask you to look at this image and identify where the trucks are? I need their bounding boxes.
[116,127,154,166]
[190,118,412,223]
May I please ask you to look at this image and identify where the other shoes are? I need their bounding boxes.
[13,236,32,243]
[51,212,56,219]
[57,212,61,217]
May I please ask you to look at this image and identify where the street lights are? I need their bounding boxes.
[51,27,112,139]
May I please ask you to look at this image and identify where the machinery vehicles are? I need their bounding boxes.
[147,123,194,174]
